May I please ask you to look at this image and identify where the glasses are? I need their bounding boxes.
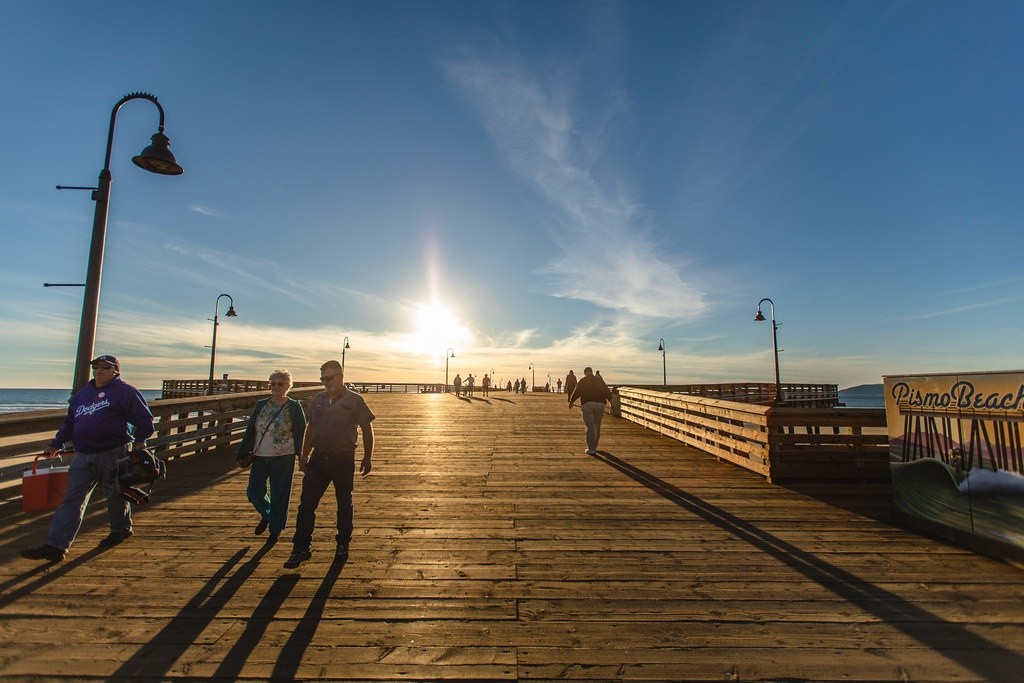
[269,382,288,387]
[92,364,116,370]
[320,373,341,383]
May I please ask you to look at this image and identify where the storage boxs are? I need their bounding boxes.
[21,465,70,512]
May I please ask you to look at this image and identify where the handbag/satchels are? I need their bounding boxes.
[239,452,254,468]
[121,448,166,506]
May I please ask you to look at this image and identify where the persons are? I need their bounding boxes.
[508,378,526,394]
[236,371,306,543]
[565,370,577,402]
[483,374,491,397]
[596,371,602,379]
[546,382,549,391]
[21,355,155,561]
[568,367,614,454]
[463,374,474,396]
[284,360,376,569]
[557,379,562,394]
[454,374,461,397]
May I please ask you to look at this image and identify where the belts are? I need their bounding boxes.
[315,452,354,459]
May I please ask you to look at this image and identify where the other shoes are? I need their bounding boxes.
[100,529,133,546]
[21,546,64,562]
[255,518,268,535]
[267,535,279,543]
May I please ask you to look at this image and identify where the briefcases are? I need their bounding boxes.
[23,453,71,512]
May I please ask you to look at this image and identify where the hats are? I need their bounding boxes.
[90,355,120,369]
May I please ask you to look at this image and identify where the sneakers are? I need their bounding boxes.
[335,544,349,559]
[585,449,596,454]
[284,547,311,568]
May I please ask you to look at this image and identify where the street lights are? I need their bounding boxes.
[342,336,351,370]
[490,369,495,387]
[754,299,784,402]
[71,92,185,388]
[528,363,534,392]
[445,348,455,385]
[657,338,667,390]
[546,372,551,386]
[207,294,238,394]
